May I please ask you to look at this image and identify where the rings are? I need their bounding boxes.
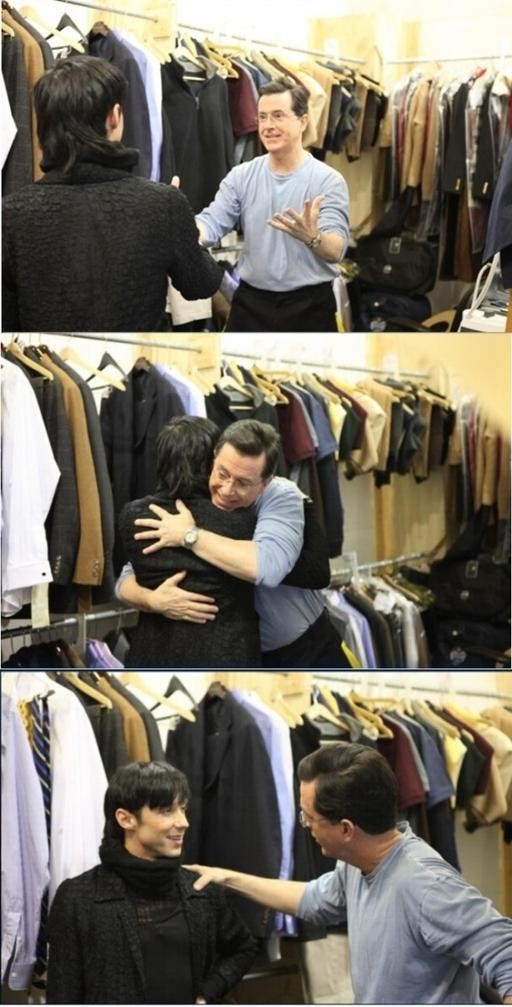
[168,576,178,583]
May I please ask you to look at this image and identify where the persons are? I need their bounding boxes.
[3,54,225,329]
[46,760,257,1003]
[180,740,511,1004]
[195,77,351,330]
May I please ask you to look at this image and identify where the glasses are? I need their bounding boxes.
[215,457,266,496]
[299,810,341,828]
[255,110,293,123]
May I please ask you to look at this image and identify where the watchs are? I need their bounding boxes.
[306,232,323,249]
[183,524,199,550]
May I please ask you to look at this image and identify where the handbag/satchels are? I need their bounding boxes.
[347,234,436,331]
[420,558,511,668]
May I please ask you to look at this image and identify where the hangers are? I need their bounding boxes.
[6,607,121,668]
[339,560,420,605]
[0,1,384,99]
[1,331,451,416]
[25,673,496,743]
